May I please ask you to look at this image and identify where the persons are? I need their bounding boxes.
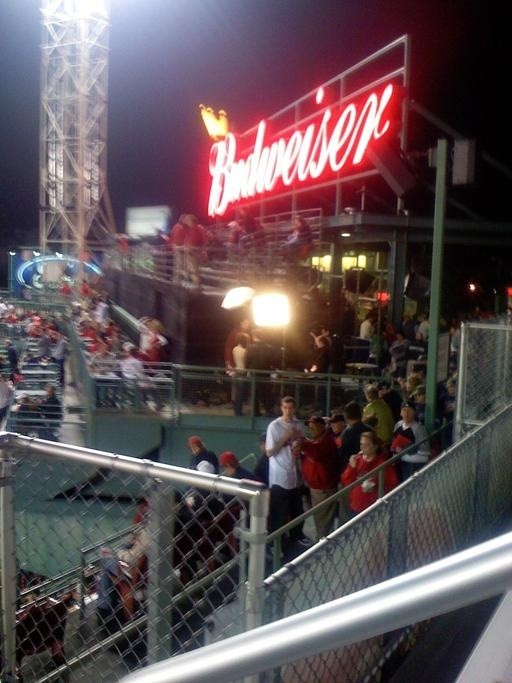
[13,583,59,629]
[168,438,257,598]
[222,289,495,559]
[112,212,314,291]
[95,516,150,629]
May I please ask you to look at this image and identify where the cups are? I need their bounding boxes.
[290,438,303,458]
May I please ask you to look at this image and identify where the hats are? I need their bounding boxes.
[411,385,426,395]
[187,435,201,446]
[122,341,137,352]
[219,452,236,466]
[327,414,344,424]
[304,415,326,426]
[257,432,265,440]
[378,381,391,389]
[401,400,414,408]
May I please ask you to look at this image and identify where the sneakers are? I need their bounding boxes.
[300,536,312,547]
